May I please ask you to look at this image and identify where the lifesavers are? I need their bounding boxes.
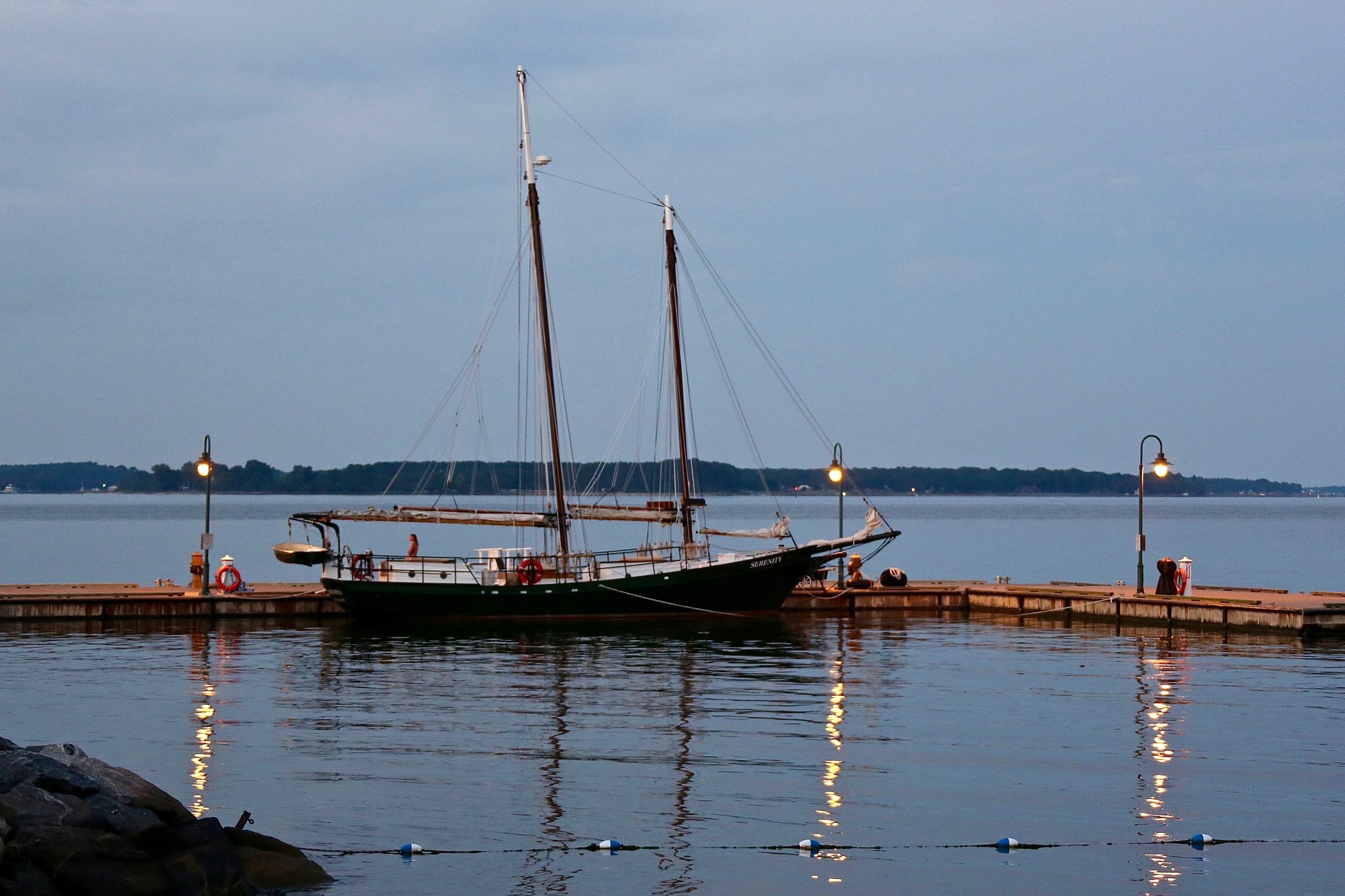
[350,554,375,580]
[517,559,543,584]
[215,565,241,592]
[1173,567,1187,595]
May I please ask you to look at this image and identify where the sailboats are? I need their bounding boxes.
[275,61,905,637]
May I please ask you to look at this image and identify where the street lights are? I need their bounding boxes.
[827,443,846,591]
[196,433,213,595]
[1136,435,1175,600]
